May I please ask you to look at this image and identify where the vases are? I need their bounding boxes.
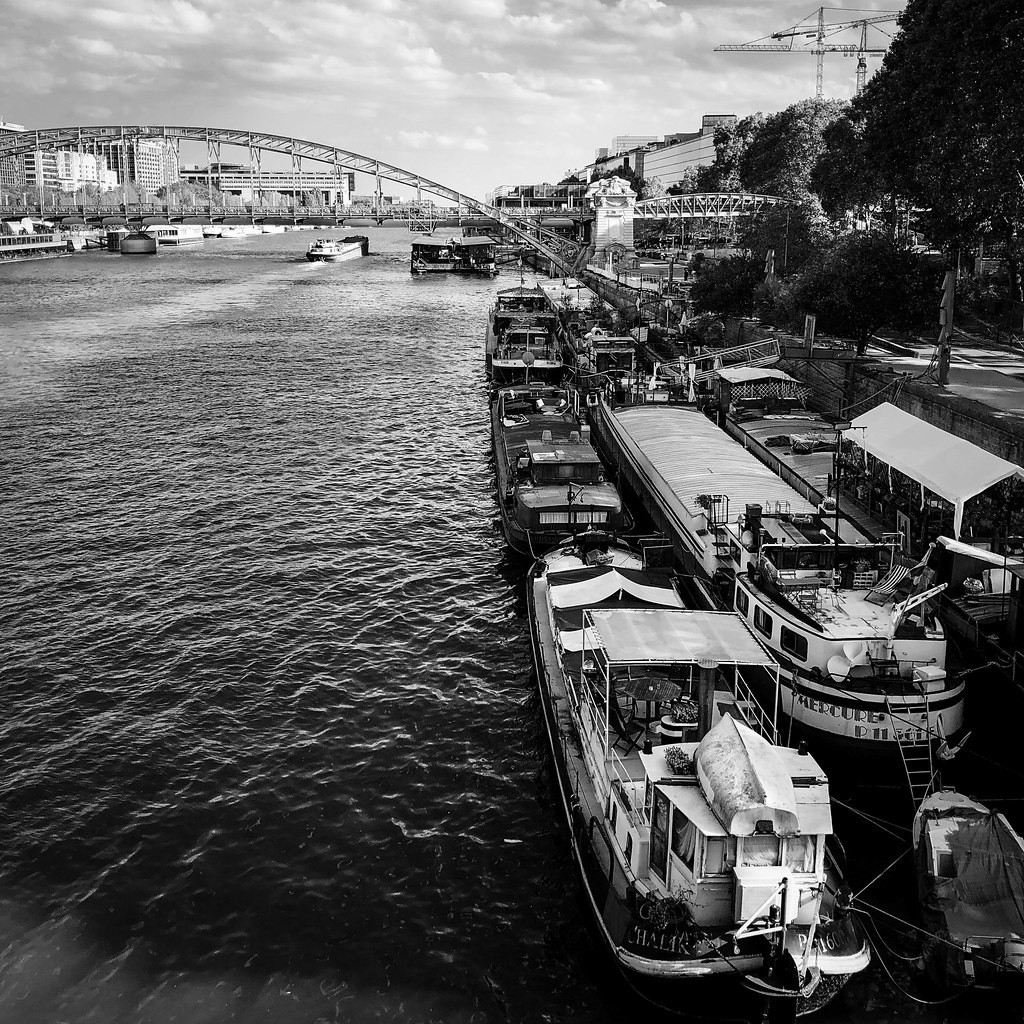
[669,763,691,775]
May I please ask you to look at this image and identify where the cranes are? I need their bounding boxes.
[713,5,903,101]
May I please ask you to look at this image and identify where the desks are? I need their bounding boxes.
[779,578,824,607]
[626,678,682,741]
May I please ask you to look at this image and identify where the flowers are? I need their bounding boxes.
[664,746,692,775]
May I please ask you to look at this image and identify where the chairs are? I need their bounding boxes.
[862,564,909,607]
[780,567,842,615]
[600,665,690,757]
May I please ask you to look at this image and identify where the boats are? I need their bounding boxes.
[484,272,1024,1024]
[305,234,370,262]
